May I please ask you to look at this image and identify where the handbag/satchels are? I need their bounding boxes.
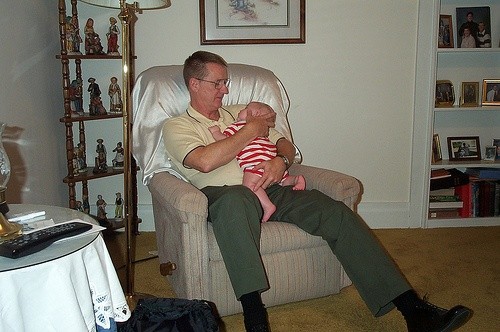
[117,298,228,332]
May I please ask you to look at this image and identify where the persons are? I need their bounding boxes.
[459,13,491,48]
[487,85,498,102]
[163,50,474,332]
[97,195,108,222]
[70,76,83,113]
[458,142,470,156]
[208,101,306,222]
[112,141,125,170]
[84,19,104,54]
[107,77,123,114]
[87,77,107,116]
[115,192,124,220]
[94,139,108,174]
[65,16,76,53]
[107,17,121,55]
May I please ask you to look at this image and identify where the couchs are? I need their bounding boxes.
[126,65,360,316]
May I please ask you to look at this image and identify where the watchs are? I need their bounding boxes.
[276,155,290,169]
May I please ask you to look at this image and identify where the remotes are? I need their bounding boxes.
[0,222,93,259]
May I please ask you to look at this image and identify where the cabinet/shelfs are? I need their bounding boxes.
[55,0,143,239]
[420,0,500,228]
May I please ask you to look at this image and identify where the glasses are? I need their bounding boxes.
[189,76,231,90]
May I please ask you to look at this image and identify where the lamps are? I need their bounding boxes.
[78,0,172,314]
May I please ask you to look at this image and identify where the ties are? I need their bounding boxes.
[494,90,496,95]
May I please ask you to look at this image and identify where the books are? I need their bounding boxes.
[430,169,500,218]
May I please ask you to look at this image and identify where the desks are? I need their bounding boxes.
[0,203,131,332]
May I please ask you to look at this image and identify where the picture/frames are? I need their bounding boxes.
[432,134,442,162]
[447,135,482,162]
[438,14,454,49]
[197,0,307,45]
[482,79,500,106]
[435,80,456,108]
[460,82,482,107]
[453,5,494,49]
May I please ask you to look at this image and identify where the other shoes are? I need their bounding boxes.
[405,291,473,332]
[244,312,272,332]
